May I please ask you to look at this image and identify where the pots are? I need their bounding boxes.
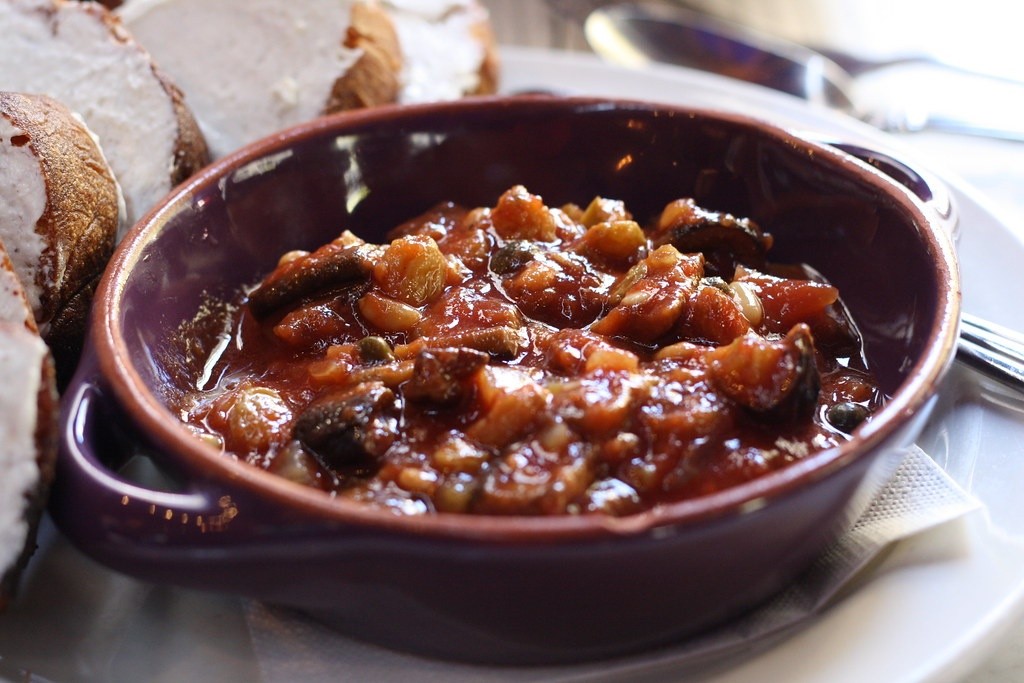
[59,99,958,667]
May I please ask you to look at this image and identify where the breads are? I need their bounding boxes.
[0,0,504,592]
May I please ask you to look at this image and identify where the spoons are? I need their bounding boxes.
[620,17,1024,144]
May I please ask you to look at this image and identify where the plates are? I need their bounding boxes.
[0,47,1024,683]
